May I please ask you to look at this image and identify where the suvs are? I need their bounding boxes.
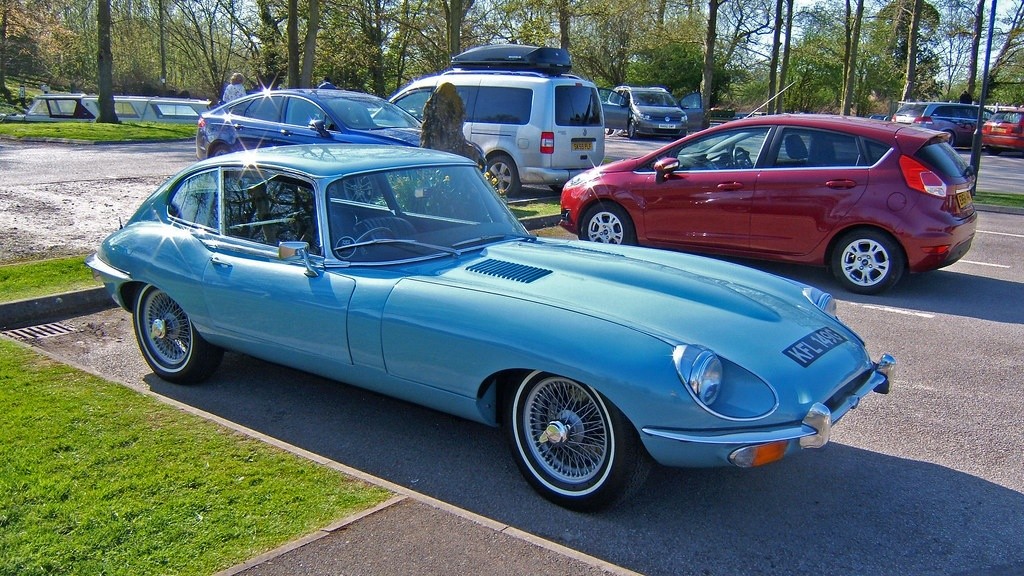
[597,86,707,142]
[890,102,996,149]
[370,43,606,200]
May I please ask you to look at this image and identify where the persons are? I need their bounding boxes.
[609,129,624,137]
[222,73,247,103]
[317,76,336,89]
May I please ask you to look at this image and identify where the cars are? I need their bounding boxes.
[559,114,978,294]
[981,108,1024,156]
[194,88,490,176]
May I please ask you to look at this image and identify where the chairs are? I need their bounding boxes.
[353,217,418,244]
[289,102,314,125]
[311,232,360,260]
[786,134,838,163]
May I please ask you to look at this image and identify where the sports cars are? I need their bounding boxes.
[85,144,896,515]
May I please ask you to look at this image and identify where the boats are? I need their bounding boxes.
[0,91,212,126]
